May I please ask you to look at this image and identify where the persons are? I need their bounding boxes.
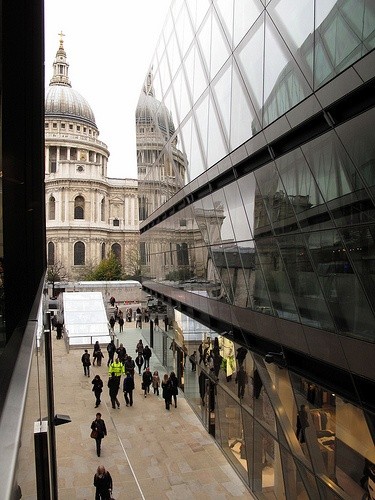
[110,296,116,307]
[153,315,160,332]
[93,464,115,500]
[180,344,188,368]
[163,315,169,331]
[92,375,103,408]
[162,374,173,410]
[123,371,135,407]
[169,339,178,357]
[81,339,160,398]
[189,336,222,412]
[89,413,107,457]
[108,304,150,333]
[107,372,121,409]
[55,320,63,341]
[294,405,308,444]
[234,346,263,400]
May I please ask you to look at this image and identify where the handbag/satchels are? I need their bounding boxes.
[90,431,98,438]
[141,383,147,390]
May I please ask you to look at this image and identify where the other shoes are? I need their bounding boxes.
[94,405,98,408]
[112,406,115,409]
[118,403,120,407]
